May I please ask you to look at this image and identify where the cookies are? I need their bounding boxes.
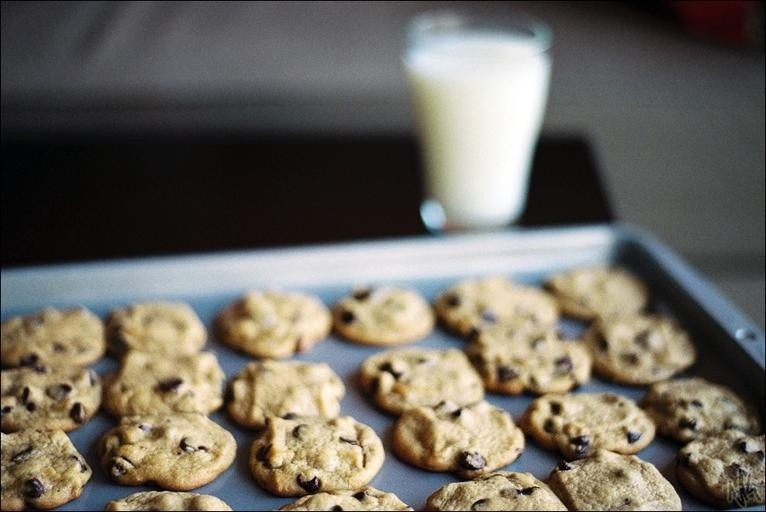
[1,266,765,512]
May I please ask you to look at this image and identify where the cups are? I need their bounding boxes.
[399,9,559,234]
